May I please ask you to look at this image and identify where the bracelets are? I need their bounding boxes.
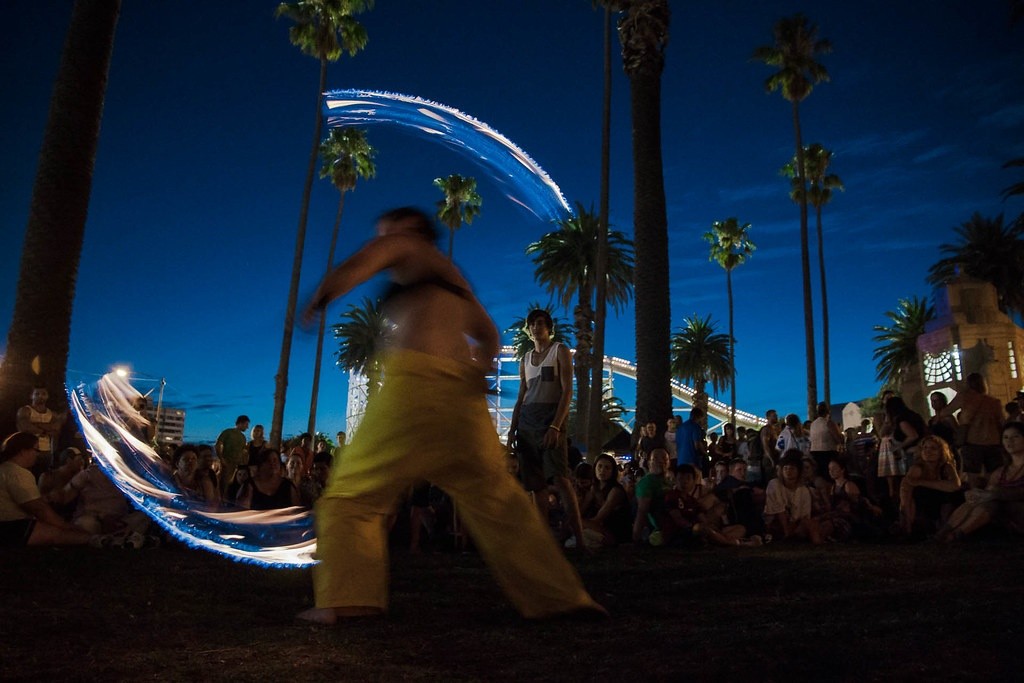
[550,424,562,433]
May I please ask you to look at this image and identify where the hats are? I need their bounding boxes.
[59,447,82,463]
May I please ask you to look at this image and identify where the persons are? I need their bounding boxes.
[297,207,611,625]
[0,369,1023,586]
[503,307,591,558]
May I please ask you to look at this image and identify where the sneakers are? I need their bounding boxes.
[737,535,763,548]
